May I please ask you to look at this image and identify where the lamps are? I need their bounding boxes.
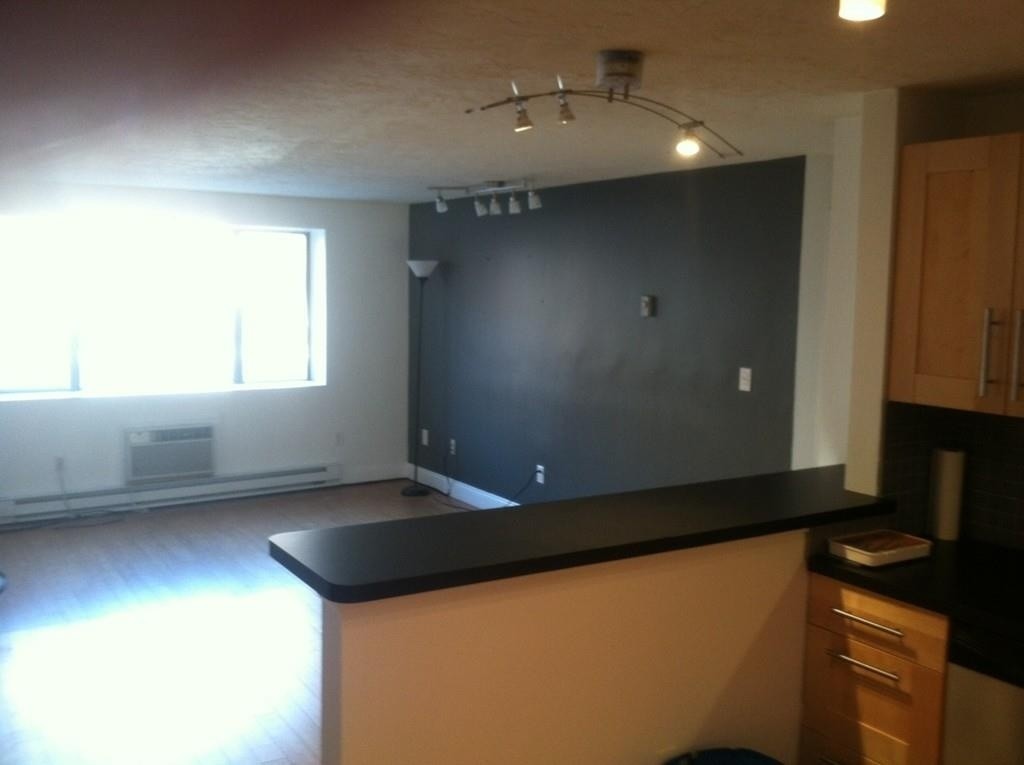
[402,260,437,496]
[463,74,748,162]
[418,176,543,218]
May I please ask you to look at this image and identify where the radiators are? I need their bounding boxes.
[124,423,216,489]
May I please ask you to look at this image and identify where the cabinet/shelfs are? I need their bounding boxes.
[877,132,1024,420]
[790,570,945,765]
